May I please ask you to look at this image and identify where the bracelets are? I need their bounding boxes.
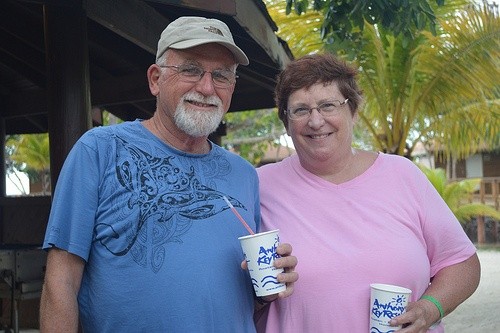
[419,295,443,326]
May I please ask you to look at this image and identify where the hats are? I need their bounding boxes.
[155,16,250,67]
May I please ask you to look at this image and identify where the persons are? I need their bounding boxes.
[248,53,482,333]
[39,16,262,333]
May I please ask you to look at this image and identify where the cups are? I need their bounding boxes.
[370,284,412,333]
[238,228,287,297]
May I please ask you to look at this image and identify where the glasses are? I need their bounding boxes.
[282,98,350,121]
[159,64,239,89]
